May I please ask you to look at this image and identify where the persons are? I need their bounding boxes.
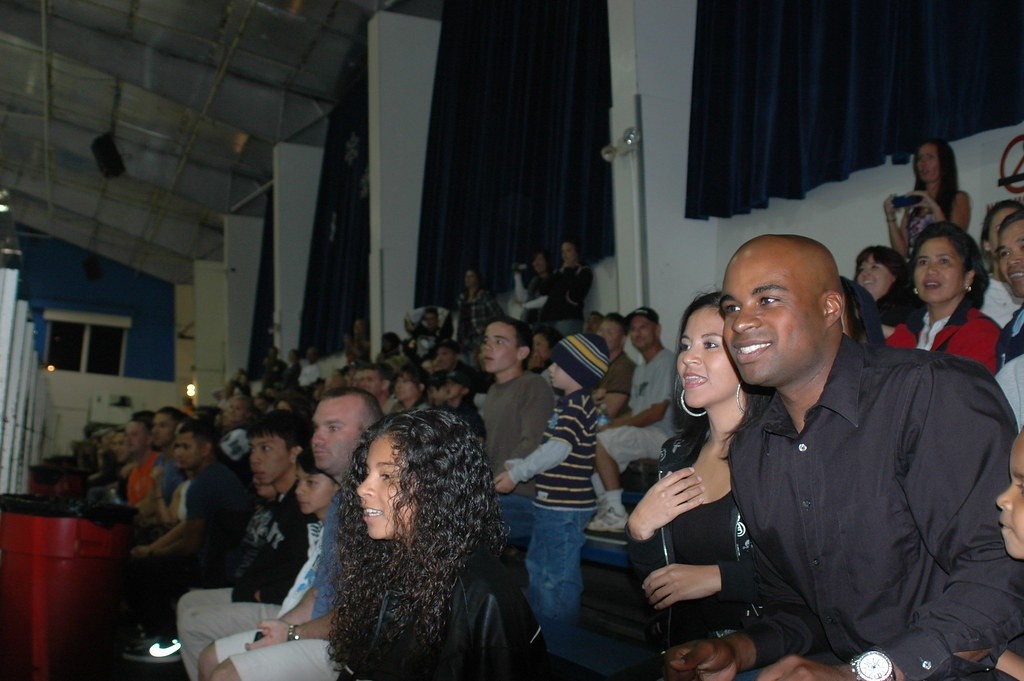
[840,137,1024,434]
[197,387,383,681]
[593,312,635,418]
[586,307,678,534]
[994,425,1024,681]
[327,408,548,681]
[494,333,609,627]
[621,292,776,645]
[477,317,556,539]
[321,240,593,445]
[73,346,324,680]
[662,233,1024,681]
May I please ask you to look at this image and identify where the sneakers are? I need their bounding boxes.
[588,506,628,532]
[123,636,181,663]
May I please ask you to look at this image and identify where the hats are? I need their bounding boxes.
[625,307,658,324]
[550,332,610,389]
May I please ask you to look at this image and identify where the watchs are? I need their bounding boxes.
[288,625,299,642]
[849,646,896,681]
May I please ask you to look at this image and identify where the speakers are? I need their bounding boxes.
[90,132,125,177]
[81,255,105,282]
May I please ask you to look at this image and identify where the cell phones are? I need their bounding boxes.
[893,194,913,208]
[253,632,264,643]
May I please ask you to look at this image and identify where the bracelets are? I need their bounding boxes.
[155,495,164,500]
[887,218,897,223]
[147,544,154,556]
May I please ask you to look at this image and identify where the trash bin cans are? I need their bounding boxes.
[28,463,82,501]
[0,493,140,681]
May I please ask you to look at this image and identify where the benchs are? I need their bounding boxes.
[530,457,661,681]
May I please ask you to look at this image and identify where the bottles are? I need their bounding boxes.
[108,488,123,504]
[597,404,610,427]
